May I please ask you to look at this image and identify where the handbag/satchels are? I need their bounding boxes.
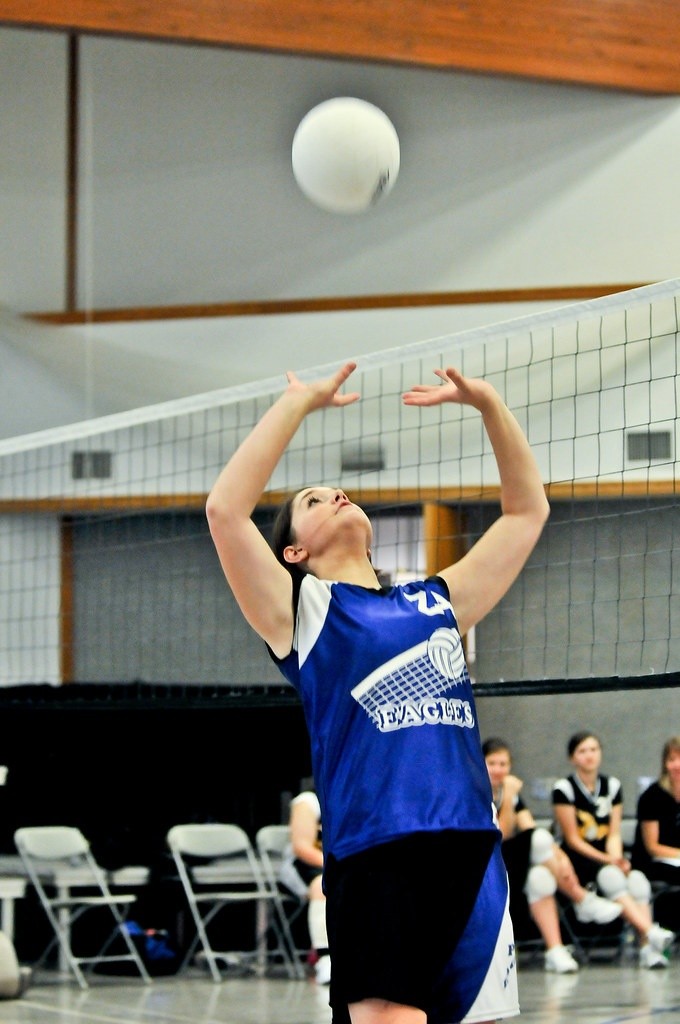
[90,921,195,977]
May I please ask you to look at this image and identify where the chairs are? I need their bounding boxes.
[166,824,296,982]
[15,826,153,990]
[256,825,312,978]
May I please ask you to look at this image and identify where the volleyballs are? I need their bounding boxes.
[290,95,402,215]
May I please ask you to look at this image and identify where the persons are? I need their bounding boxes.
[205,361,551,1024]
[553,731,675,969]
[631,737,680,902]
[481,737,623,974]
[283,790,331,985]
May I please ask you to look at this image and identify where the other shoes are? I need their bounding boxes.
[542,943,580,974]
[314,955,332,987]
[573,891,623,924]
[646,923,676,953]
[639,944,669,969]
